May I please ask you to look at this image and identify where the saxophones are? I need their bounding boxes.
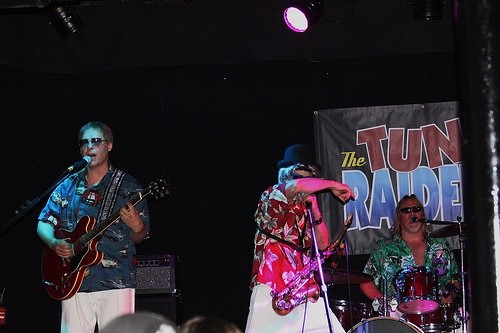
[270,212,354,318]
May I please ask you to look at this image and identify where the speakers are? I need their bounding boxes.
[134,297,177,326]
[135,255,176,295]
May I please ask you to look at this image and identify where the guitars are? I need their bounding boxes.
[37,173,170,301]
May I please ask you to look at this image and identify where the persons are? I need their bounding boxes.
[359,195,471,333]
[37,122,152,333]
[244,144,348,333]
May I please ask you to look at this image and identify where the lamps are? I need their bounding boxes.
[283,0,325,33]
[33,0,80,38]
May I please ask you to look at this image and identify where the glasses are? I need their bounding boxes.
[78,138,107,146]
[399,205,423,214]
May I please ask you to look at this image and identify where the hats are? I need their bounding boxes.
[277,144,322,172]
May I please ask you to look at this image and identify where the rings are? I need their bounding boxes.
[122,213,125,217]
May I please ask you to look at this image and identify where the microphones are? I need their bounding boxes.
[411,217,419,223]
[67,156,92,173]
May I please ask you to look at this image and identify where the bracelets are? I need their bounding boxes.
[313,214,323,226]
[134,223,147,235]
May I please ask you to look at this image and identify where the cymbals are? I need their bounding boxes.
[428,221,469,240]
[328,268,373,285]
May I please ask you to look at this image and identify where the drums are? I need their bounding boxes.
[346,315,424,333]
[329,299,372,332]
[394,305,449,333]
[400,267,440,315]
